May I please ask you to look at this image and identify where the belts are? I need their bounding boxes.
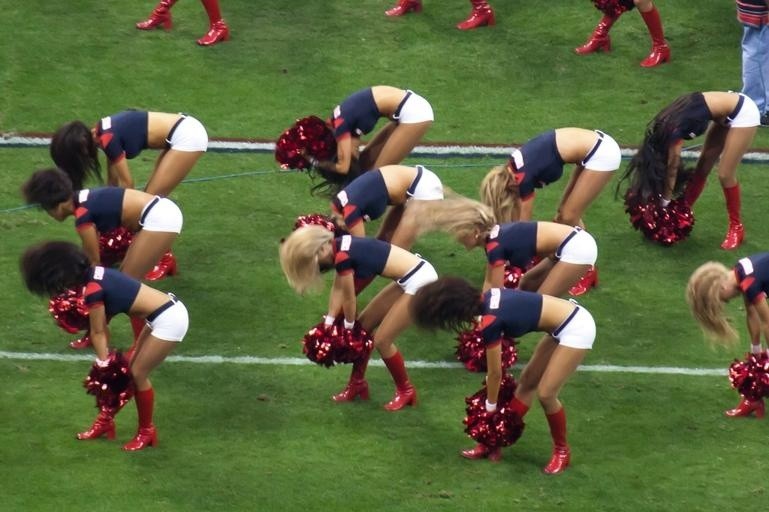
[552,299,579,336]
[406,165,423,197]
[556,225,582,260]
[165,113,189,145]
[725,89,746,126]
[145,291,181,322]
[395,89,414,116]
[398,261,426,284]
[136,194,162,223]
[583,128,603,164]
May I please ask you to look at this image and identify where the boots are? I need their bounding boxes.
[333,328,374,403]
[639,5,673,69]
[725,395,765,418]
[457,0,495,31]
[75,388,117,445]
[385,0,425,18]
[719,182,745,251]
[459,397,531,463]
[379,349,420,412]
[70,311,146,364]
[679,175,708,209]
[194,1,233,47]
[120,388,161,453]
[134,0,179,33]
[145,246,179,281]
[572,1,627,54]
[541,406,572,474]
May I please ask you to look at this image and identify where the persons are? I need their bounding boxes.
[329,164,444,295]
[19,240,190,451]
[407,186,598,298]
[384,0,496,30]
[575,0,671,68]
[21,169,184,357]
[136,0,229,46]
[408,275,597,475]
[612,91,761,250]
[479,128,622,296]
[50,110,209,282]
[280,225,439,412]
[686,251,769,419]
[299,86,433,199]
[736,1,769,126]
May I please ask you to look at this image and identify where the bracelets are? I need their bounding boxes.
[95,358,110,368]
[484,398,497,412]
[343,318,355,330]
[752,344,761,353]
[659,197,671,207]
[324,316,335,326]
[308,156,319,167]
